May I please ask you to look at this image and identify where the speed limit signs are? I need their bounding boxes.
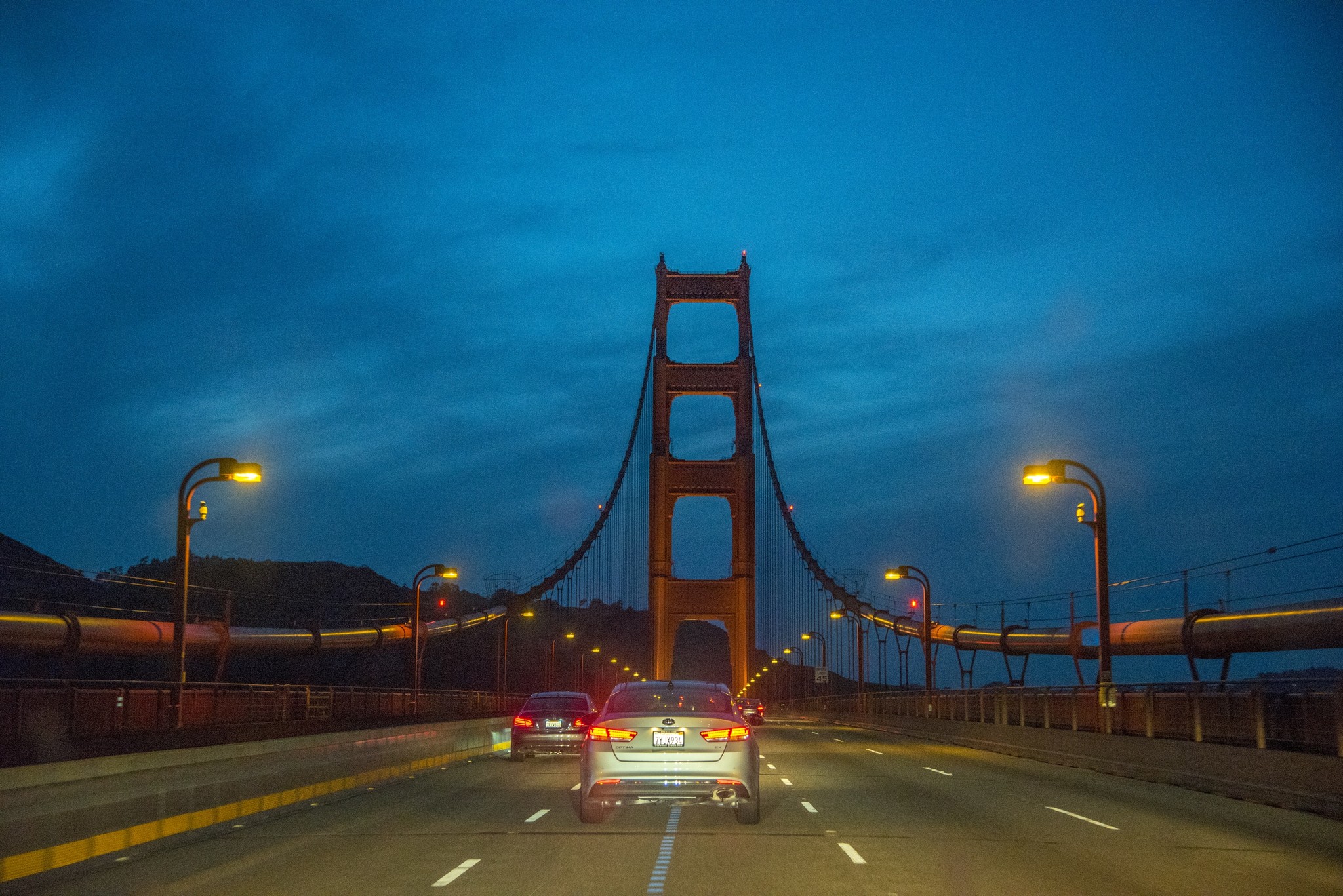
[814,666,828,683]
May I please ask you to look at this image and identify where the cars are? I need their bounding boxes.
[580,679,765,825]
[509,692,601,762]
[734,697,764,719]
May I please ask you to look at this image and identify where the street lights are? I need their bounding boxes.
[600,657,639,701]
[168,456,264,733]
[830,608,863,713]
[802,630,828,698]
[495,610,536,713]
[1022,458,1111,734]
[577,646,602,693]
[413,564,458,724]
[884,565,932,717]
[547,631,575,691]
[754,647,805,699]
